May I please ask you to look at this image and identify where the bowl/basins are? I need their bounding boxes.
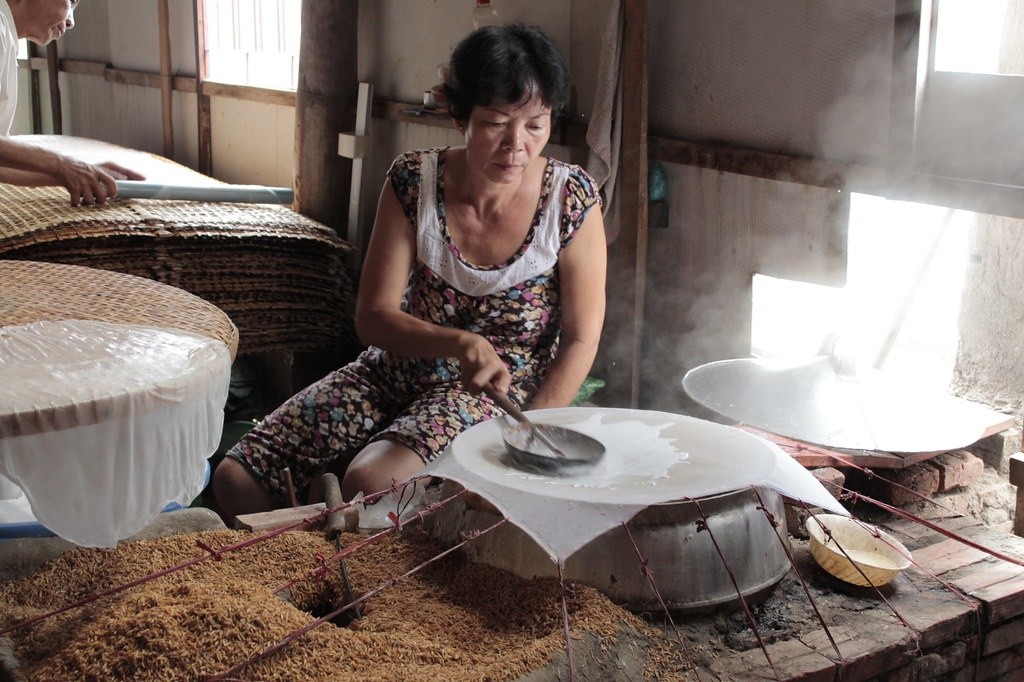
[504,421,606,473]
[806,514,914,588]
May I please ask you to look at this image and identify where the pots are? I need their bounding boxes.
[433,407,795,612]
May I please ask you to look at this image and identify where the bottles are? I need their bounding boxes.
[473,0,497,30]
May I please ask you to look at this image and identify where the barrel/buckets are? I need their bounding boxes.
[0,457,213,539]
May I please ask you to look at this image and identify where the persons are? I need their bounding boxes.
[214,24,606,522]
[0,0,145,206]
